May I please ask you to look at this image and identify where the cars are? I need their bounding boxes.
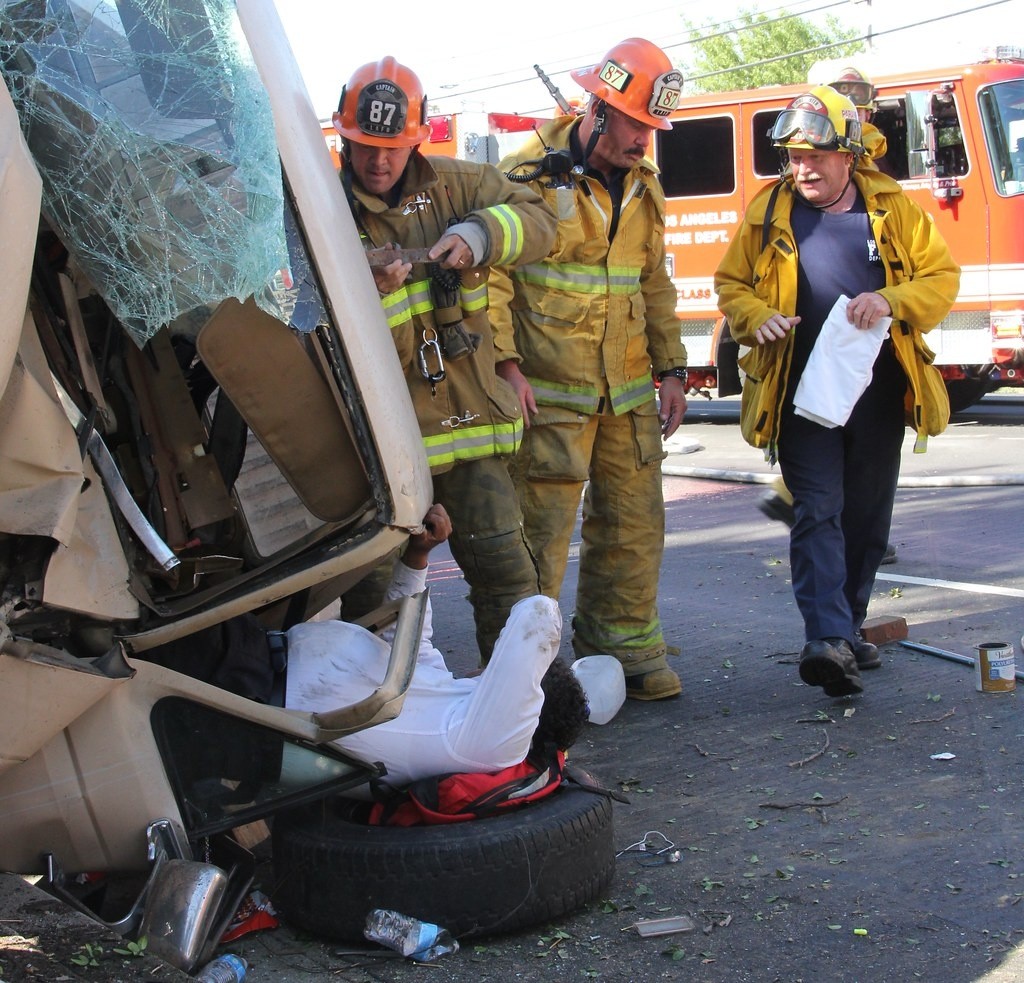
[2,0,434,977]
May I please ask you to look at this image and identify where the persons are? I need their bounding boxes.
[712,66,962,696]
[331,55,562,675]
[147,502,592,788]
[489,36,688,702]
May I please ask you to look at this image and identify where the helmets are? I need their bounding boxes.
[569,38,684,130]
[332,56,431,148]
[773,86,870,156]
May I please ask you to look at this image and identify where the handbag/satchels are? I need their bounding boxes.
[361,744,630,829]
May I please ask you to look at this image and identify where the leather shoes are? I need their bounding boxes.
[848,639,883,669]
[800,638,865,696]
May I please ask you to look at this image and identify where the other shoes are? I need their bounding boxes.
[626,666,682,700]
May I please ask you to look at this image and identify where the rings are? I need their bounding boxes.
[459,259,465,265]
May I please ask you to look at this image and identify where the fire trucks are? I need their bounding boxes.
[316,44,1024,422]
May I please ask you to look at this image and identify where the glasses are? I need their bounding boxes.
[773,109,837,149]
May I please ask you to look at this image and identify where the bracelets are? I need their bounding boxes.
[656,366,686,380]
